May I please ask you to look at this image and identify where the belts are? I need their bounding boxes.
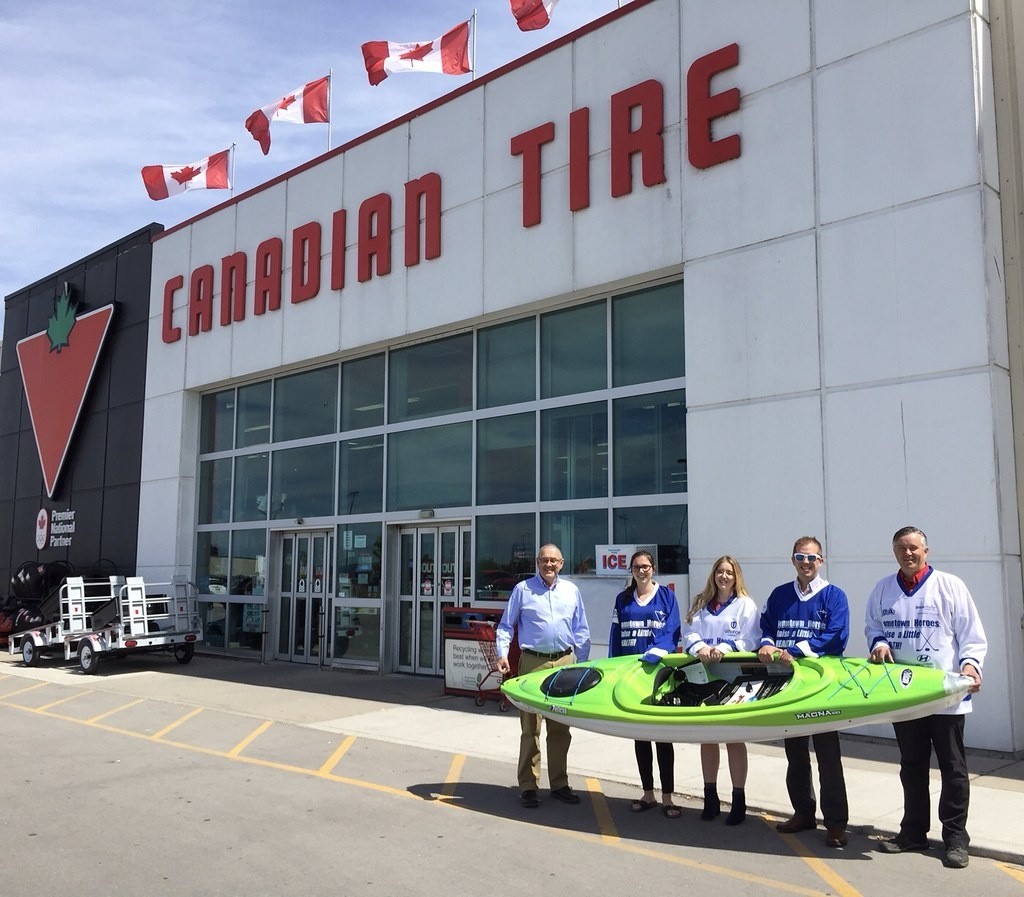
[522,647,573,661]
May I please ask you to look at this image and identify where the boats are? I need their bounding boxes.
[501,648,977,742]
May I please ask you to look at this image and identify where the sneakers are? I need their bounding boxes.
[878,830,929,853]
[944,845,970,868]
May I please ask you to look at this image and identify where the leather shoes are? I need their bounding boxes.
[520,790,542,808]
[825,828,848,848]
[551,785,580,803]
[776,814,817,833]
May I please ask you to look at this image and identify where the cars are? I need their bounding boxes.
[478,568,536,622]
[207,574,358,659]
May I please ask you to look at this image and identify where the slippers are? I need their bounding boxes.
[660,804,682,818]
[631,799,658,812]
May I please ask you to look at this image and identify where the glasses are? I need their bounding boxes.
[538,557,563,566]
[714,569,736,576]
[793,552,822,563]
[631,564,652,572]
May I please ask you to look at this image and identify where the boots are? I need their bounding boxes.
[701,782,721,821]
[725,787,747,826]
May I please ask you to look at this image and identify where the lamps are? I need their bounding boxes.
[420,510,434,519]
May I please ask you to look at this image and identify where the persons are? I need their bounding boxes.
[608,549,682,819]
[758,535,851,847]
[681,554,762,826]
[496,543,592,810]
[864,526,988,869]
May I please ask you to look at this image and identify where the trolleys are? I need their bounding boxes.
[466,619,521,712]
